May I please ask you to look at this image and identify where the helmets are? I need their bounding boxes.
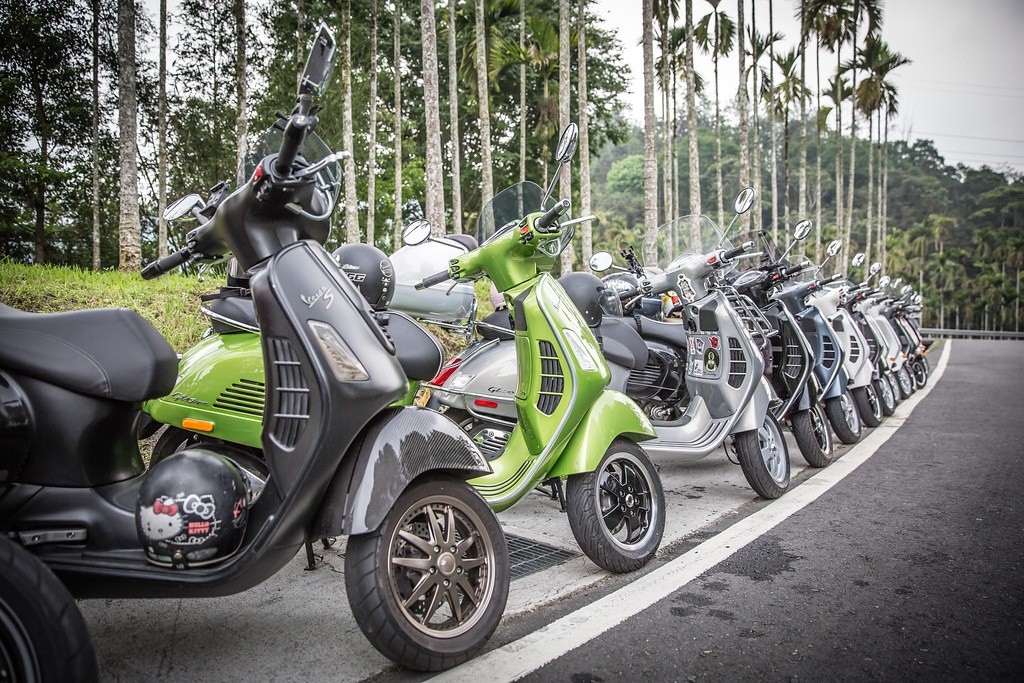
[660,291,685,317]
[558,272,623,328]
[135,441,253,570]
[601,270,642,315]
[331,243,396,311]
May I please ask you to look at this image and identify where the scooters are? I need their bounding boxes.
[384,186,930,497]
[0,18,511,682]
[139,118,668,573]
